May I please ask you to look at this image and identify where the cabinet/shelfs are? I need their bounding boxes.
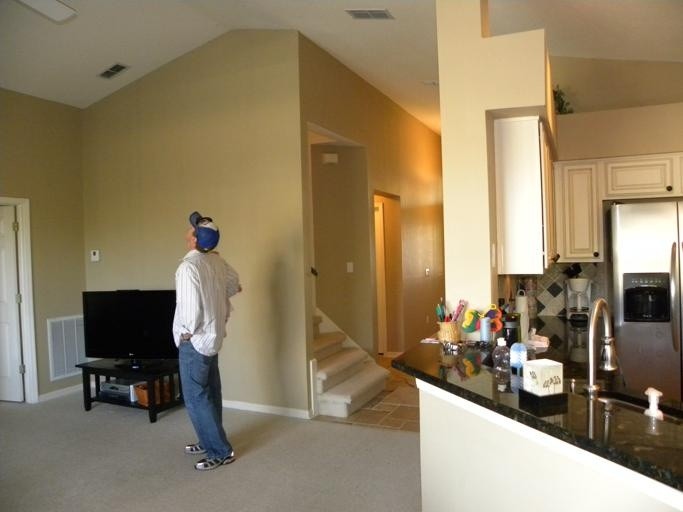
[492,117,556,275]
[555,160,604,264]
[604,152,683,202]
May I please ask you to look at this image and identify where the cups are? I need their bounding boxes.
[437,346,460,368]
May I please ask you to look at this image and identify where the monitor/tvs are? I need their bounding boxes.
[82,289,179,369]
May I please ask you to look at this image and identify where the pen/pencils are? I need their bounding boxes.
[436,297,465,321]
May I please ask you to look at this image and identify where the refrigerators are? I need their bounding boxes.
[610,200,682,413]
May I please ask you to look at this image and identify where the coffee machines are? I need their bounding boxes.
[565,322,593,364]
[563,277,592,322]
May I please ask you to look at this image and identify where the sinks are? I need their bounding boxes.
[594,397,683,425]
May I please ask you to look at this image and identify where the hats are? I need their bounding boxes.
[190,212,219,251]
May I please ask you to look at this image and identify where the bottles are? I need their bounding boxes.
[493,371,510,393]
[503,320,518,347]
[493,337,510,372]
[515,289,529,346]
[479,316,491,343]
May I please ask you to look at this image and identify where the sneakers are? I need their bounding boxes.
[185,443,207,454]
[194,451,236,470]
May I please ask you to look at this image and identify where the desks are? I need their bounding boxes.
[75,357,183,423]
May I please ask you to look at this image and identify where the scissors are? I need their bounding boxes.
[478,341,488,349]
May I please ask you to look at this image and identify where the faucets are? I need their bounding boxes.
[583,402,616,455]
[583,297,618,399]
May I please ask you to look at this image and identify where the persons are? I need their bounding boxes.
[172,211,242,471]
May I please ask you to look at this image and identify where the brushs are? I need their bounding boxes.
[510,343,528,394]
